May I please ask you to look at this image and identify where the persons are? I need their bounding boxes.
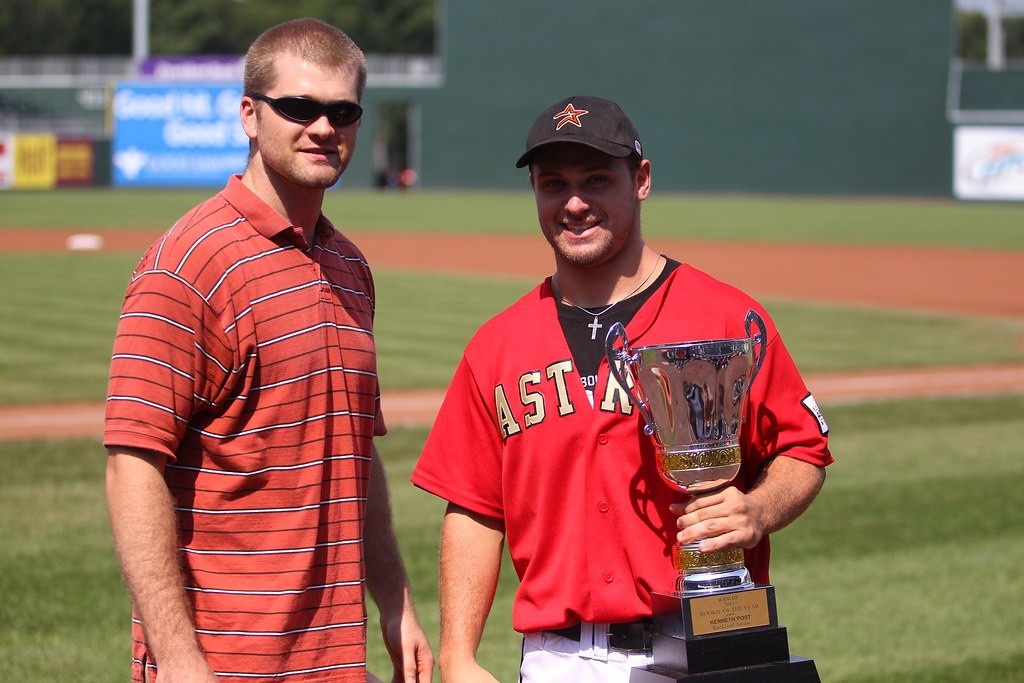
[102,21,433,682]
[410,94,837,683]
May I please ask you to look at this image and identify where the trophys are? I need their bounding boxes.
[605,310,823,683]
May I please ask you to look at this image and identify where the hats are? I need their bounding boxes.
[515,96,643,162]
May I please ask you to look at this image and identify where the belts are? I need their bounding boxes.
[549,620,653,652]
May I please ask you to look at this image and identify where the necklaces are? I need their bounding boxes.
[550,253,660,341]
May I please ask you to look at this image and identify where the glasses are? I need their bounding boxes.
[244,92,364,127]
